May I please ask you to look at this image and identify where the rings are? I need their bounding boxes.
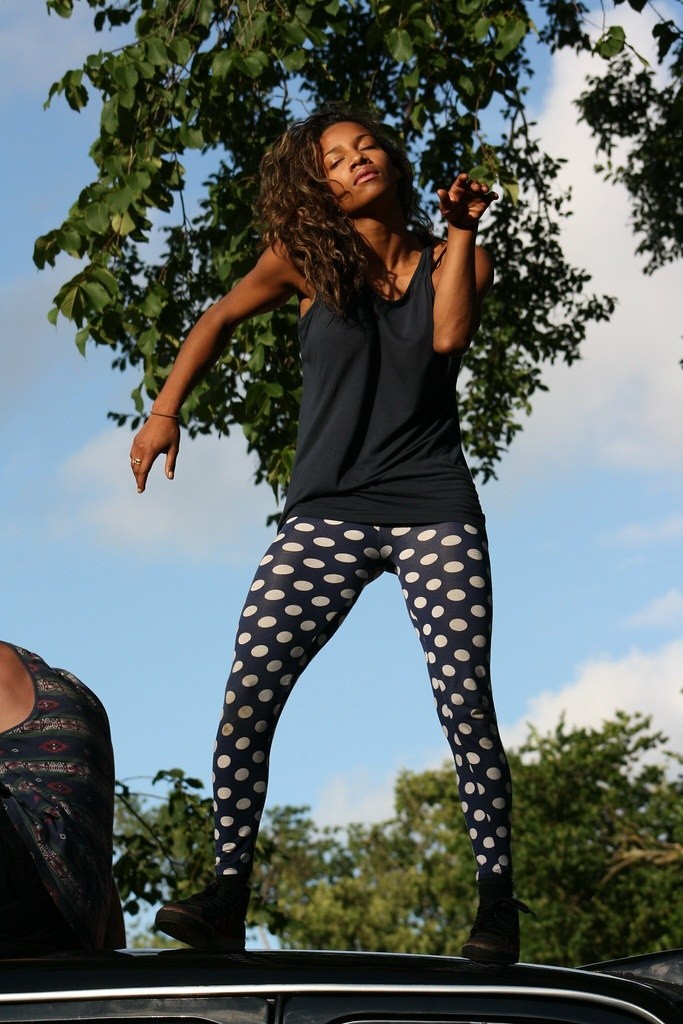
[132,457,140,464]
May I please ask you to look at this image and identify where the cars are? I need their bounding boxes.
[0,947,683,1024]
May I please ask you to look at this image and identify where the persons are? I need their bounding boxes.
[129,107,529,966]
[0,636,127,963]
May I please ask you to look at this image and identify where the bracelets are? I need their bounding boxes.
[150,411,181,419]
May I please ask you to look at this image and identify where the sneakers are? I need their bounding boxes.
[155,874,251,954]
[461,899,537,964]
